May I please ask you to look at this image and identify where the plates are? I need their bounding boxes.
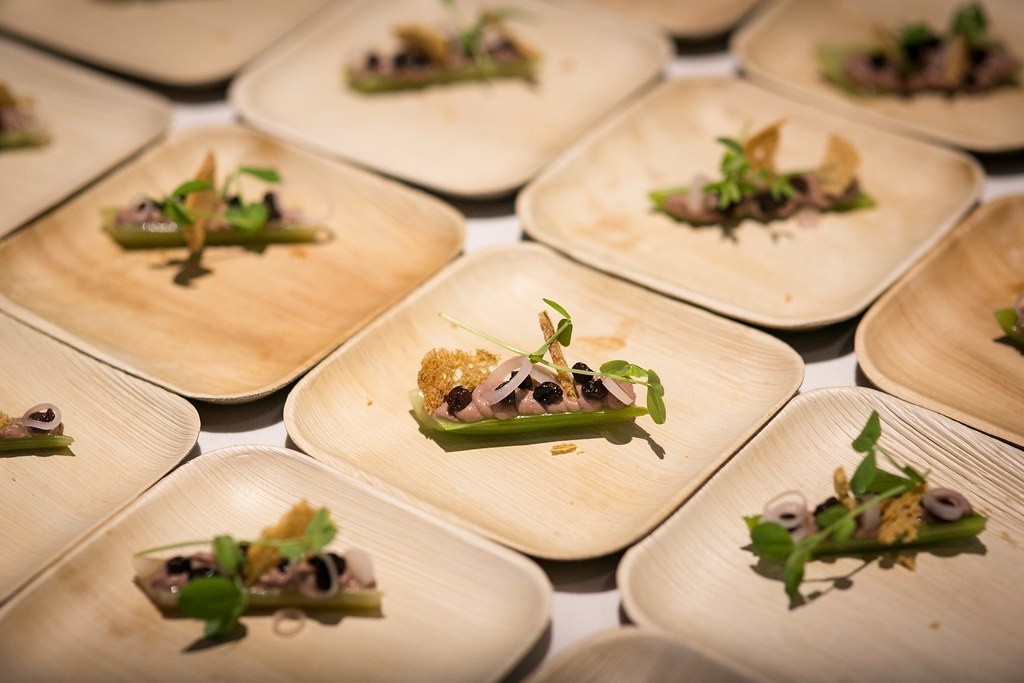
[0,1,1024,683]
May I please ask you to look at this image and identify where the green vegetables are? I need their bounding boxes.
[809,0,1024,97]
[743,407,988,609]
[345,10,539,92]
[647,134,876,226]
[131,504,384,642]
[410,298,667,443]
[100,165,322,247]
